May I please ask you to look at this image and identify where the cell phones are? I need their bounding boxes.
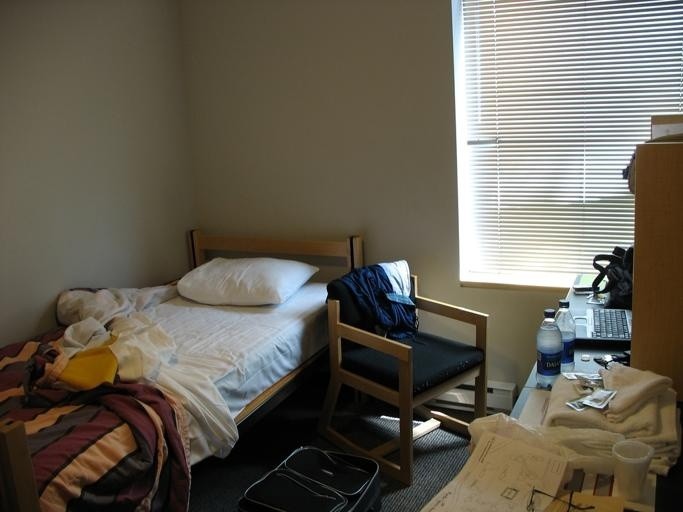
[572,284,599,290]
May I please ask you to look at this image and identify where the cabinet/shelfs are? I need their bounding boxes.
[630,143,683,401]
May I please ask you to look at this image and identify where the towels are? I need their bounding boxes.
[540,365,682,477]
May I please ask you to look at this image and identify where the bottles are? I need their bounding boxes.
[539,299,575,392]
[647,113,683,141]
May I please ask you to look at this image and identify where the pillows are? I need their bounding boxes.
[176,257,319,306]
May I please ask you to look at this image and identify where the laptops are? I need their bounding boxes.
[586,307,632,340]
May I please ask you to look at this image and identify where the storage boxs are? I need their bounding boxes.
[650,115,683,142]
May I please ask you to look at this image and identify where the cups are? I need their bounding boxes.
[612,442,656,503]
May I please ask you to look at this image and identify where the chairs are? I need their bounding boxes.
[317,274,490,486]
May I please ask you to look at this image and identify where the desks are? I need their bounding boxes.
[509,288,682,511]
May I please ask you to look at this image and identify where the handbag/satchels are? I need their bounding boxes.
[591,246,633,310]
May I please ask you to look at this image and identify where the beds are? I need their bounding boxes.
[0,229,364,512]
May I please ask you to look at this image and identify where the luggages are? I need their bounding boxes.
[236,444,382,512]
